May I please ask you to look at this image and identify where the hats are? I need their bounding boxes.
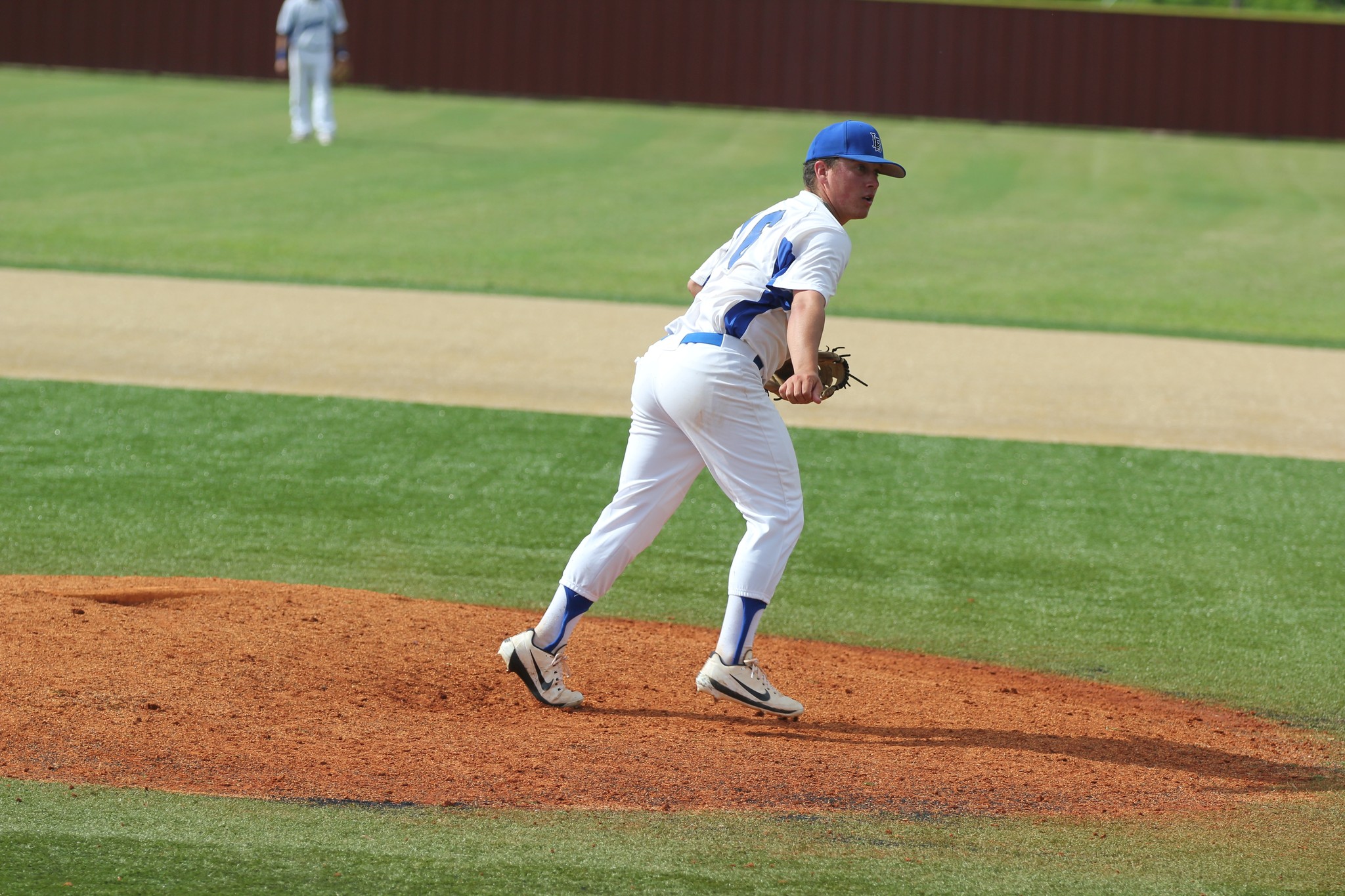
[805,120,906,178]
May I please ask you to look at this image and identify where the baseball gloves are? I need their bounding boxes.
[764,350,850,401]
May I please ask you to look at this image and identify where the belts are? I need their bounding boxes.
[663,333,764,370]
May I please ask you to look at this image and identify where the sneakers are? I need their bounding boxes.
[695,649,804,721]
[497,628,584,707]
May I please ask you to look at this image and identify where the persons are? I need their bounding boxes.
[497,120,907,720]
[273,0,349,146]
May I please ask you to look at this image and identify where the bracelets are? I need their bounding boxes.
[275,50,287,59]
